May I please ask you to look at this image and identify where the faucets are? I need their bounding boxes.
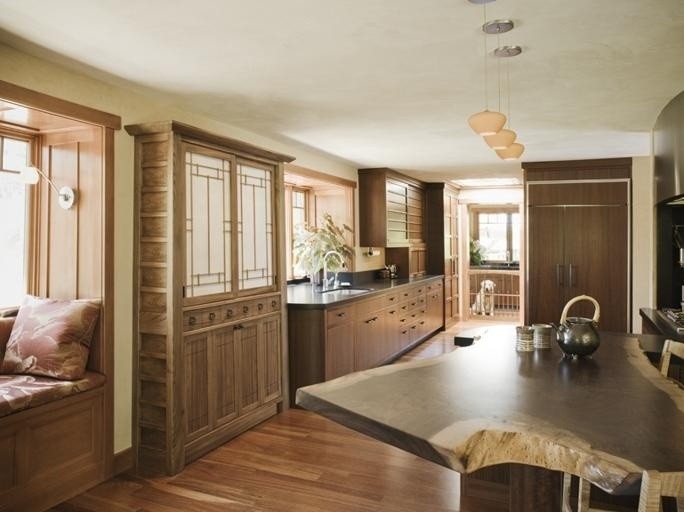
[321,250,345,291]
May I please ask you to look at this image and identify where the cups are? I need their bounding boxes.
[516,325,534,351]
[532,324,552,350]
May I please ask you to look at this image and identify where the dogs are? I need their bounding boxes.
[468,278,497,317]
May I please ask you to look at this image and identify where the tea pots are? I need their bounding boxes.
[548,294,601,360]
[383,263,401,279]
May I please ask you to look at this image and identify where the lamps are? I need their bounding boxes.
[466,1,507,137]
[483,18,519,152]
[495,143,524,160]
[22,166,78,211]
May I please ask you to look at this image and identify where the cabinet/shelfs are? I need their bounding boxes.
[385,248,428,278]
[324,274,444,382]
[523,167,632,335]
[123,119,297,476]
[358,167,426,248]
[425,182,460,331]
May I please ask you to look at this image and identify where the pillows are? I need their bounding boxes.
[1,295,103,382]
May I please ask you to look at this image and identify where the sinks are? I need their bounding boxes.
[316,287,373,296]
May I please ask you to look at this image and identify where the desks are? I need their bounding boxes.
[293,322,682,510]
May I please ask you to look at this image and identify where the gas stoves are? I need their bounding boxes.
[656,306,684,338]
